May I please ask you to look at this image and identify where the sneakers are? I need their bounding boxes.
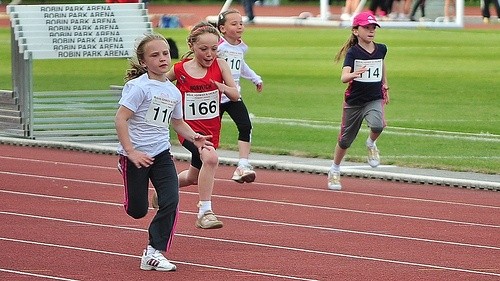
[194,209,225,229]
[231,164,256,184]
[327,170,342,190]
[367,143,380,167]
[152,193,159,207]
[140,249,177,272]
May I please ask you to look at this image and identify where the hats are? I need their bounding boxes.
[352,12,380,29]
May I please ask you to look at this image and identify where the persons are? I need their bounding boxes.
[390,0,406,19]
[368,0,392,17]
[482,0,500,23]
[328,13,388,190]
[165,20,240,230]
[341,0,361,21]
[213,9,263,184]
[241,0,256,24]
[444,0,456,22]
[112,34,215,271]
[408,0,425,21]
